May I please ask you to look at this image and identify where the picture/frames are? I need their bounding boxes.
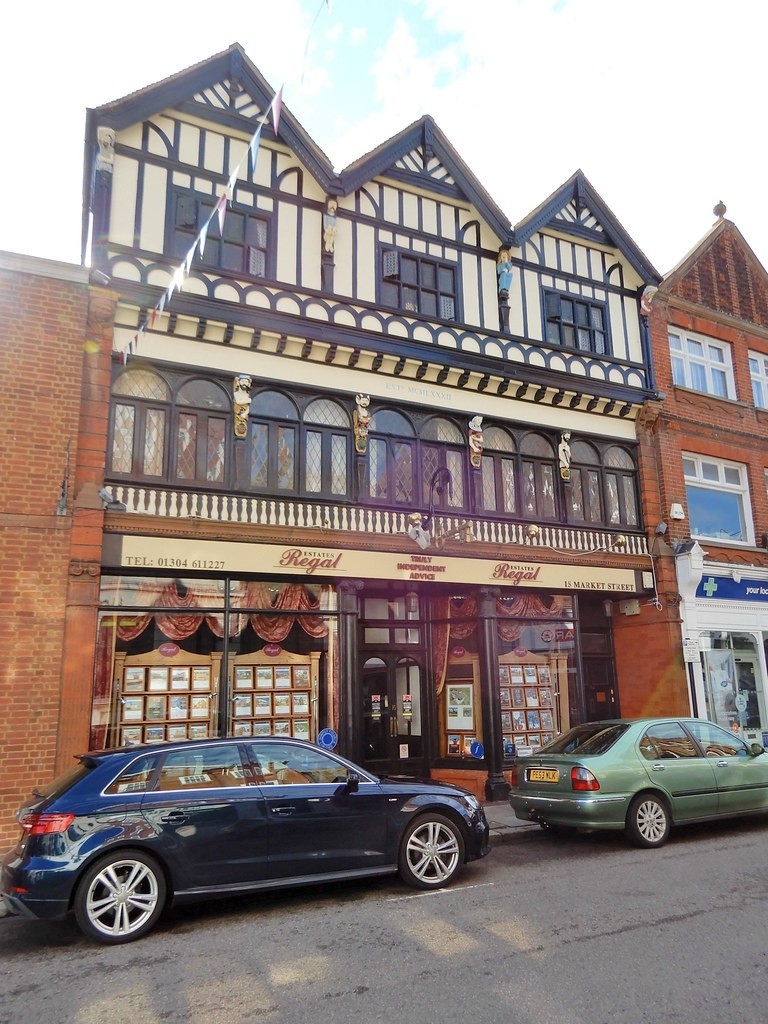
[122,694,213,720]
[503,733,554,746]
[501,710,553,730]
[124,666,213,691]
[121,723,208,746]
[499,665,552,684]
[233,718,310,740]
[445,684,476,731]
[233,692,312,717]
[235,665,310,689]
[463,735,476,755]
[448,734,462,755]
[500,687,553,708]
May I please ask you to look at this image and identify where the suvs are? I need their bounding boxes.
[1,733,492,947]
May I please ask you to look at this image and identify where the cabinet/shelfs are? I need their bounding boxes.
[498,646,558,739]
[113,643,224,747]
[228,642,321,745]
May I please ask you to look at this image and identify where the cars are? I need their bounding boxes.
[508,716,768,848]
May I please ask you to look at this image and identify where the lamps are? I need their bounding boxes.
[593,534,626,551]
[396,512,422,541]
[406,584,418,612]
[509,525,539,545]
[448,533,476,543]
[602,598,614,617]
